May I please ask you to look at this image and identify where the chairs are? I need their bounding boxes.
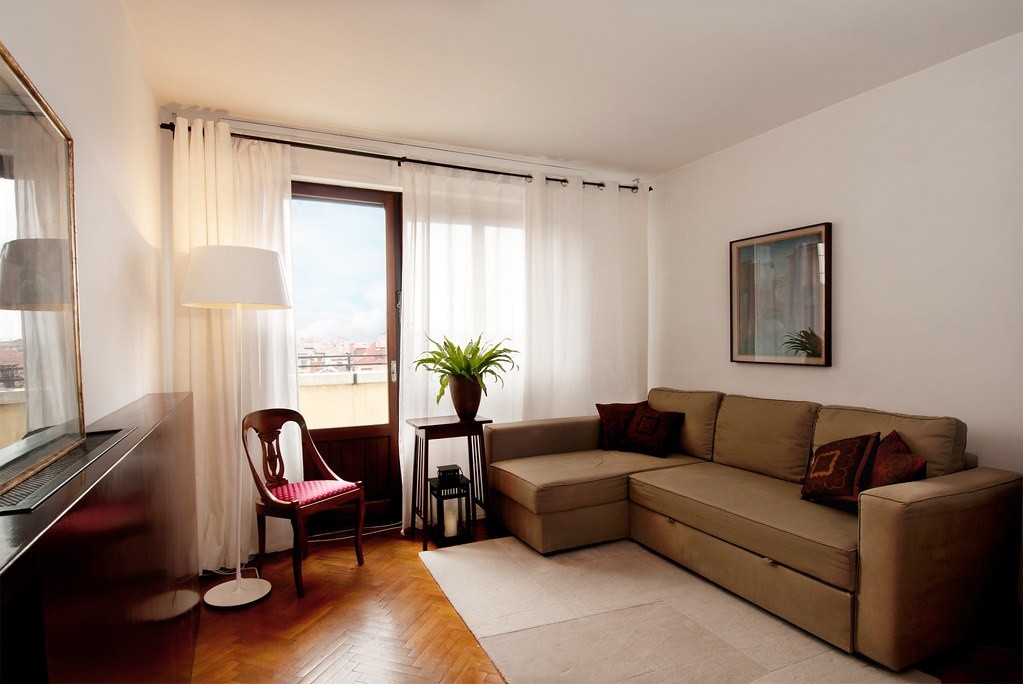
[241,408,367,599]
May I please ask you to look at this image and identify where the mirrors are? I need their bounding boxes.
[0,40,87,497]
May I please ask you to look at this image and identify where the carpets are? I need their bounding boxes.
[416,536,946,684]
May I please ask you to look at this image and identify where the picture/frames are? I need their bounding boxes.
[729,222,832,368]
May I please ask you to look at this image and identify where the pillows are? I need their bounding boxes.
[800,429,927,512]
[595,400,686,458]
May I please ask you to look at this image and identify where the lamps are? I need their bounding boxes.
[0,239,74,435]
[179,246,295,612]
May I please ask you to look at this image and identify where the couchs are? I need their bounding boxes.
[484,387,1023,674]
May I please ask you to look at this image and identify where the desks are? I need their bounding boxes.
[405,415,493,552]
[428,473,472,548]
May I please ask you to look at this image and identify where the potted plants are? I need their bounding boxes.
[781,327,824,365]
[412,332,521,421]
